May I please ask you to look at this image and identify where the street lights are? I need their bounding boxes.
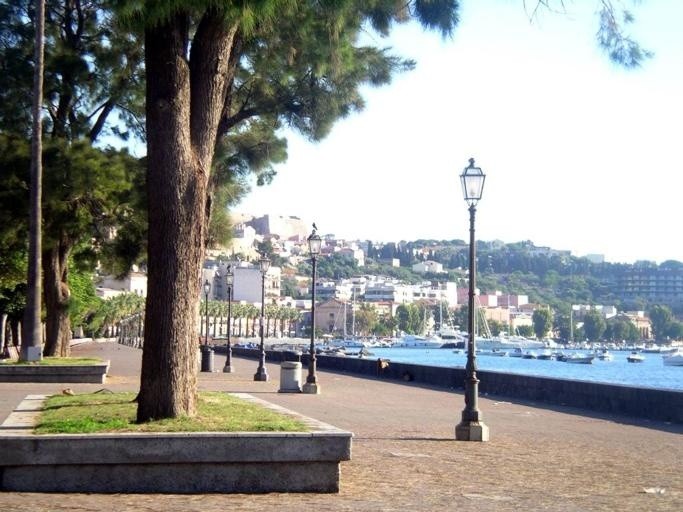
[119,309,145,349]
[203,252,270,381]
[302,229,323,394]
[456,157,489,442]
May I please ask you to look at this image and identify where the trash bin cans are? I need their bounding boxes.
[280,361,303,393]
[202,351,214,372]
